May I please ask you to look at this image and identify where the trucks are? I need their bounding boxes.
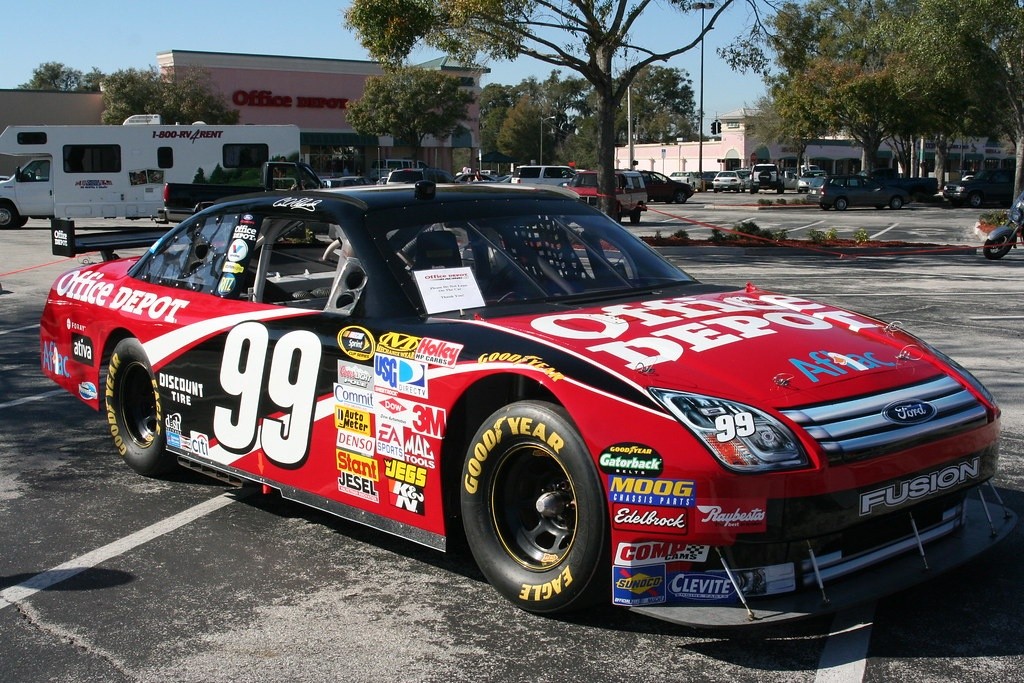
[0,115,301,229]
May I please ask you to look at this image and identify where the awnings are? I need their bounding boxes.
[300,132,379,147]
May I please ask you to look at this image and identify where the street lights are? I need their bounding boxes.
[692,1,716,177]
[539,115,555,166]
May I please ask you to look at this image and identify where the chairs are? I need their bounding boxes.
[416,230,462,269]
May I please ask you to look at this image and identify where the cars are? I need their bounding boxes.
[322,163,694,205]
[40,179,1018,643]
[798,170,826,193]
[703,165,822,193]
[819,176,911,211]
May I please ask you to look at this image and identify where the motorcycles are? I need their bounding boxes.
[983,191,1023,259]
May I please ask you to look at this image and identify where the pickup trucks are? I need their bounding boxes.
[564,171,648,225]
[151,161,325,223]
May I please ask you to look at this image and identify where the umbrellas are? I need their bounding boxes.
[476,151,515,174]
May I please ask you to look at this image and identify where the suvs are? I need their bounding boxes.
[943,167,1014,208]
[749,164,785,195]
[669,171,704,193]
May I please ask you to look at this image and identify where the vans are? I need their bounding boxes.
[371,159,430,182]
